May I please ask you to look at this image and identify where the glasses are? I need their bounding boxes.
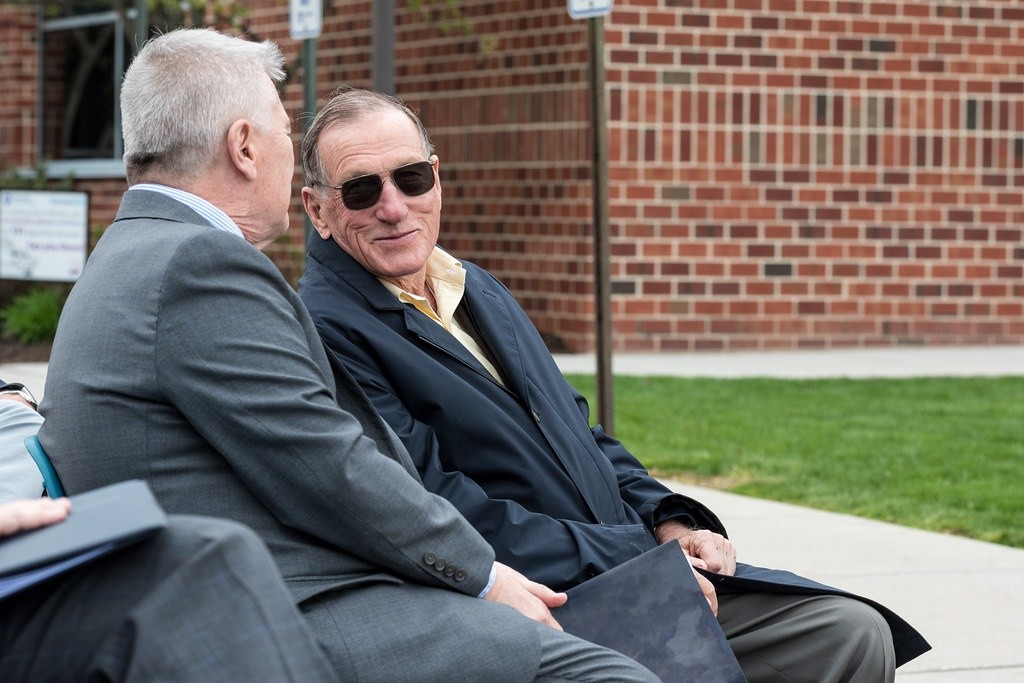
[310,159,436,211]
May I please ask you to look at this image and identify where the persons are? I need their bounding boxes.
[36,28,662,683]
[0,379,343,683]
[300,88,931,683]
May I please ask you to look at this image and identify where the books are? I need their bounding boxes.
[547,538,747,683]
[0,480,170,601]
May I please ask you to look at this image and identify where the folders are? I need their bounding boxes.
[0,477,171,599]
